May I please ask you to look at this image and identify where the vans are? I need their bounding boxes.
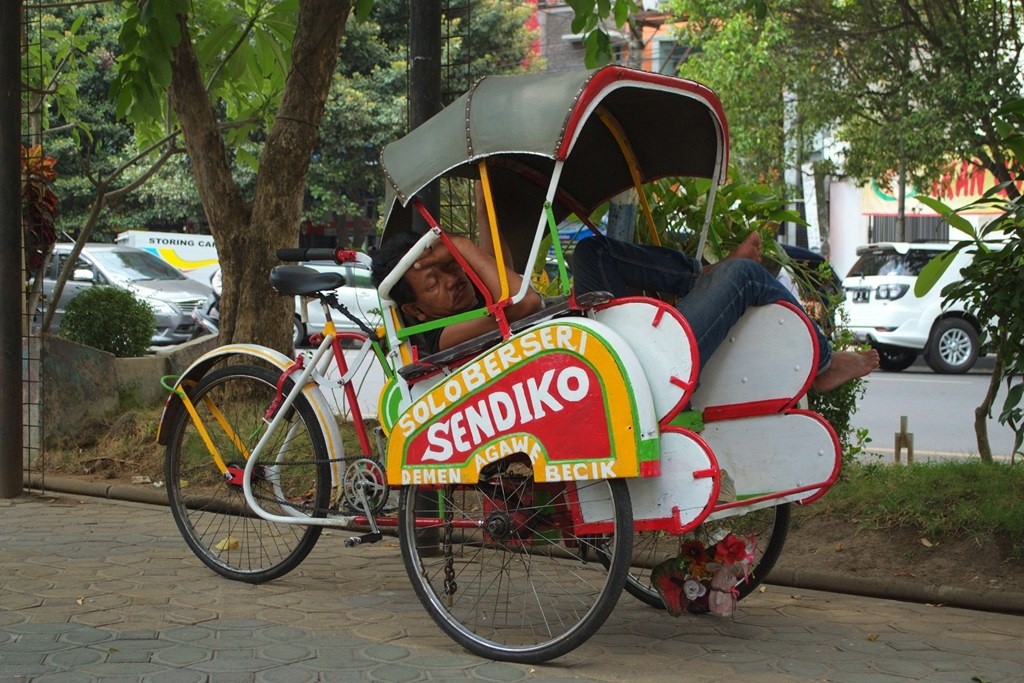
[28,244,218,344]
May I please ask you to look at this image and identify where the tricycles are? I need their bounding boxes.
[155,63,843,665]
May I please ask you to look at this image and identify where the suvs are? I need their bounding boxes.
[833,237,1024,374]
[292,261,384,350]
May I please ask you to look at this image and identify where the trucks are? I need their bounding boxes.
[117,229,222,287]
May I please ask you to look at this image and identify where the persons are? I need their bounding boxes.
[369,223,881,396]
